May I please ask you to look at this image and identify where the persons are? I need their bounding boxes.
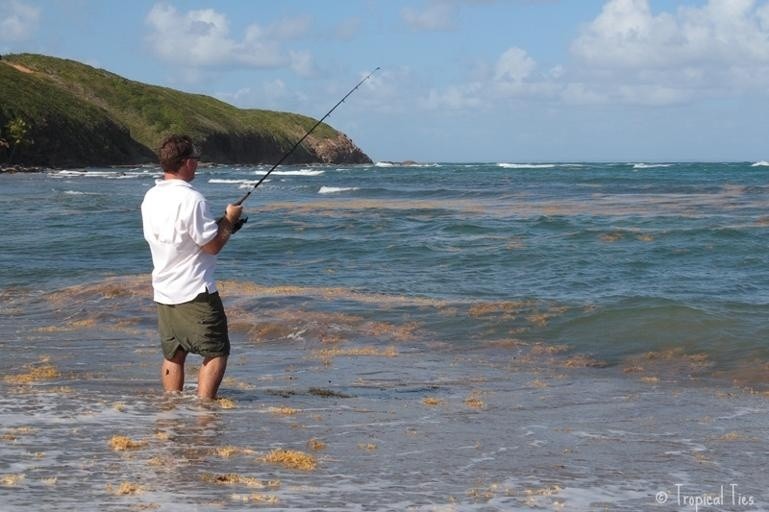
[141,133,243,398]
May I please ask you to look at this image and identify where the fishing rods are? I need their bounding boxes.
[230,68,379,234]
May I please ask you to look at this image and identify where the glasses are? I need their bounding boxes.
[176,151,201,164]
